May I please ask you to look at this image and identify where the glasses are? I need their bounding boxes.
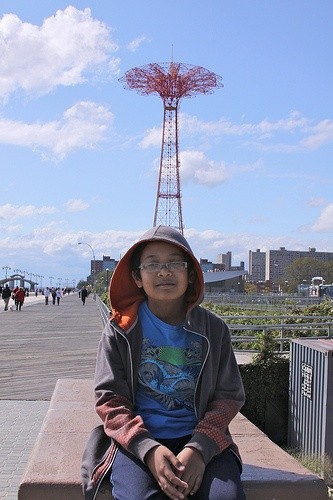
[139,261,188,272]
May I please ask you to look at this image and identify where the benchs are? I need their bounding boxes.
[17,379,330,500]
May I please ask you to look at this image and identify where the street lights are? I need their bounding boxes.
[106,267,109,286]
[20,271,76,293]
[2,266,11,283]
[14,268,21,285]
[78,242,96,301]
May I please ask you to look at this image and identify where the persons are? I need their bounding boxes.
[0,284,26,311]
[81,286,87,305]
[81,225,247,500]
[44,287,70,305]
